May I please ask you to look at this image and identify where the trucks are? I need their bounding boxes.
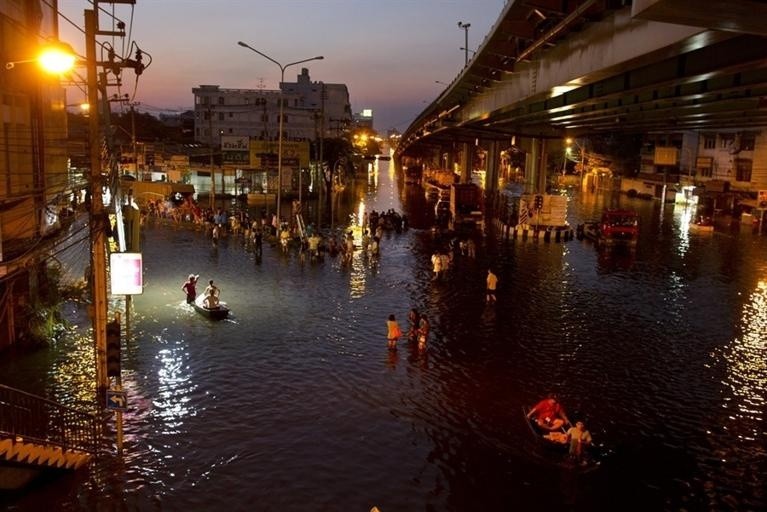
[576,207,638,248]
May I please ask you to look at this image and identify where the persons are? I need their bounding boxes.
[417,315,431,352]
[431,234,476,280]
[526,393,571,430]
[564,420,595,462]
[204,279,220,297]
[179,273,200,306]
[407,308,419,340]
[386,315,399,351]
[139,198,355,275]
[485,269,498,301]
[202,291,220,308]
[362,207,409,268]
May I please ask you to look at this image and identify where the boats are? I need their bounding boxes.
[191,299,231,321]
[522,403,601,476]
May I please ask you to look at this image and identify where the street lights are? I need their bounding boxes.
[236,40,325,241]
[458,20,475,64]
[566,136,585,190]
[3,40,78,79]
[67,101,90,113]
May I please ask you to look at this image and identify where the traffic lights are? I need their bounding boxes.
[364,157,391,161]
